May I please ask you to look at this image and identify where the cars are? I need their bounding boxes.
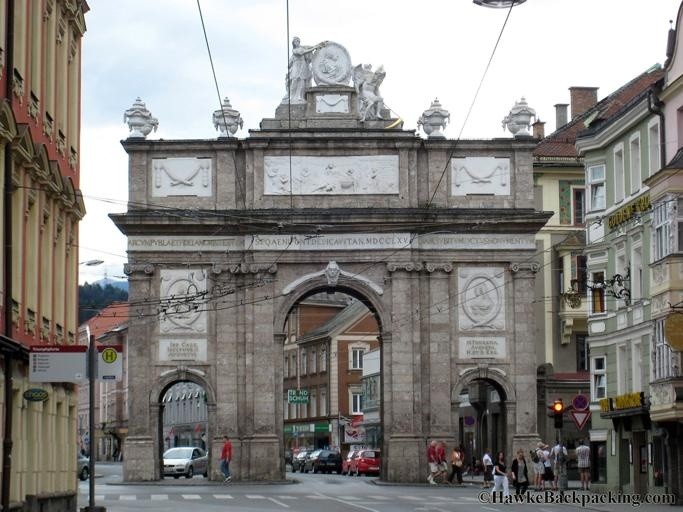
[75,447,91,482]
[283,445,381,479]
[162,445,208,479]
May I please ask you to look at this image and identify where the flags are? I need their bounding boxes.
[168,426,174,437]
[193,423,201,433]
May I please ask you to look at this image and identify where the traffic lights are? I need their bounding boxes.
[553,400,564,429]
[546,404,554,418]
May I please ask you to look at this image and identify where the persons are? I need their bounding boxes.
[433,439,449,484]
[532,441,546,491]
[490,450,509,497]
[218,435,232,482]
[482,447,493,488]
[549,438,569,489]
[352,64,384,123]
[459,445,465,463]
[575,438,592,490]
[463,458,483,477]
[541,443,557,491]
[447,445,465,486]
[510,449,529,495]
[283,36,326,105]
[425,439,441,485]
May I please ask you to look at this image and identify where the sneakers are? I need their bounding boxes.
[482,486,493,489]
[427,477,467,485]
[224,476,231,482]
[462,471,469,475]
[534,487,559,492]
[580,487,590,491]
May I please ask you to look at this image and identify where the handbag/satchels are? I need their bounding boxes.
[558,453,565,463]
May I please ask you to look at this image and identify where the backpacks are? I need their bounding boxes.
[530,450,540,463]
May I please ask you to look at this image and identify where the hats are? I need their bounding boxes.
[538,441,549,449]
